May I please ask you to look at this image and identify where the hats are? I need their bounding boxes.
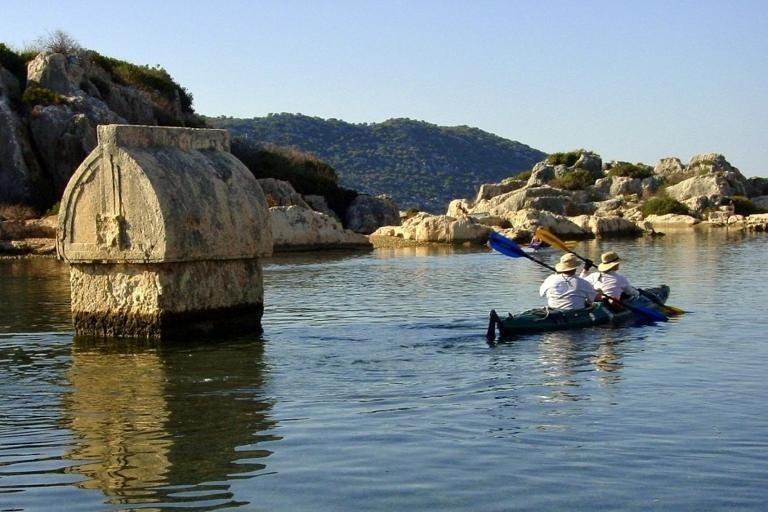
[555,252,583,273]
[598,251,623,272]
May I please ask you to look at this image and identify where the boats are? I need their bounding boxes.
[482,284,673,349]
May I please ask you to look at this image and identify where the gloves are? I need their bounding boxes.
[637,289,643,295]
[584,259,593,270]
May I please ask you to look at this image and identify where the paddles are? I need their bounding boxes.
[489,232,671,324]
[534,230,685,311]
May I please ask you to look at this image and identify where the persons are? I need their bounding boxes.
[530,226,551,249]
[539,253,603,310]
[579,251,639,302]
